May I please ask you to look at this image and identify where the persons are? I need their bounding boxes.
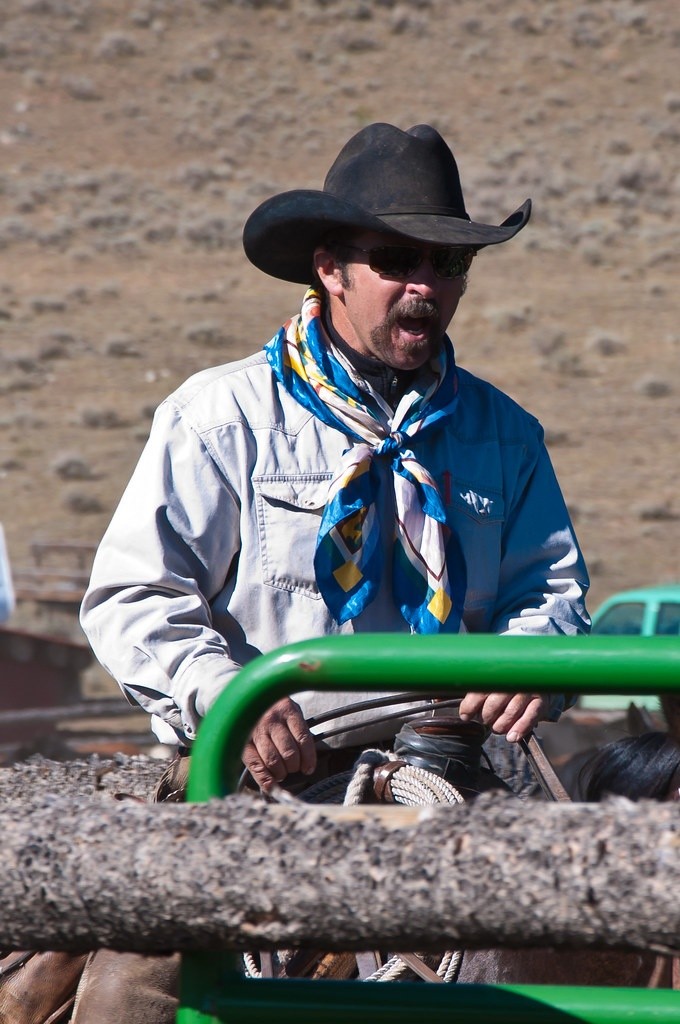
[78,121,591,792]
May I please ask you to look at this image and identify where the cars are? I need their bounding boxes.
[568,584,680,707]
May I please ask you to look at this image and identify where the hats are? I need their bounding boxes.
[241,123,532,285]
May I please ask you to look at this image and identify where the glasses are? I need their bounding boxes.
[341,239,477,280]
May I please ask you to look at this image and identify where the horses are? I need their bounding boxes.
[0,701,680,1024]
